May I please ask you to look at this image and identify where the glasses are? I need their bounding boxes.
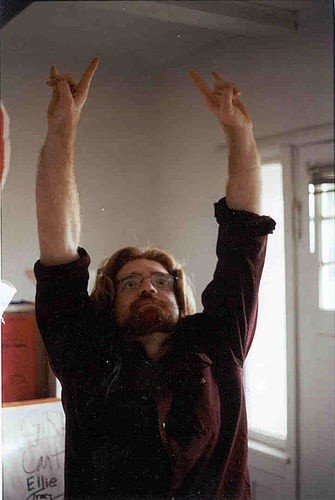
[114,272,175,289]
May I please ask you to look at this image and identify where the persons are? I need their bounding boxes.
[29,57,275,500]
